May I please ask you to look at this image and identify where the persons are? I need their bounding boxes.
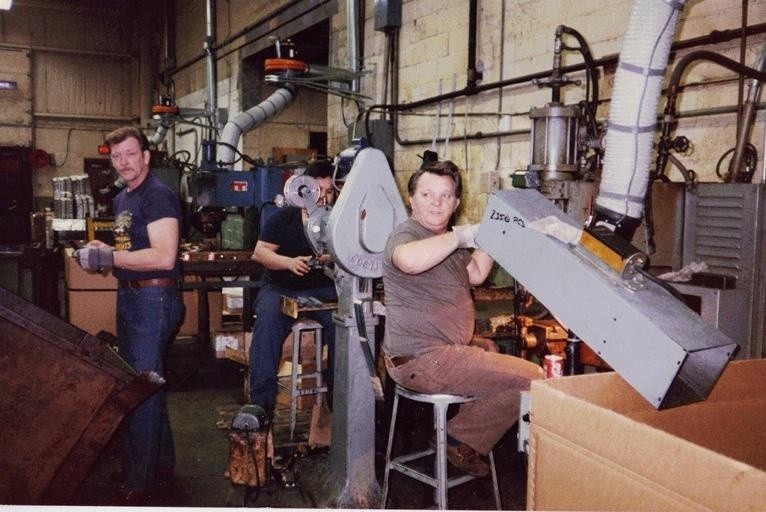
[72,126,184,505]
[381,161,548,478]
[230,157,335,433]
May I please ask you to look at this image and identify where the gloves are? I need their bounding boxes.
[452,224,480,249]
[71,245,116,271]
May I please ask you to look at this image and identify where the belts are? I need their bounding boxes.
[384,356,416,368]
[117,277,178,288]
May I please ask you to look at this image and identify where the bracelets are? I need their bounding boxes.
[120,250,128,270]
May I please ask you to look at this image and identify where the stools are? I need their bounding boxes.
[380,386,503,510]
[272,316,330,440]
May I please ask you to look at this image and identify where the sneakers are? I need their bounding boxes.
[429,427,490,477]
